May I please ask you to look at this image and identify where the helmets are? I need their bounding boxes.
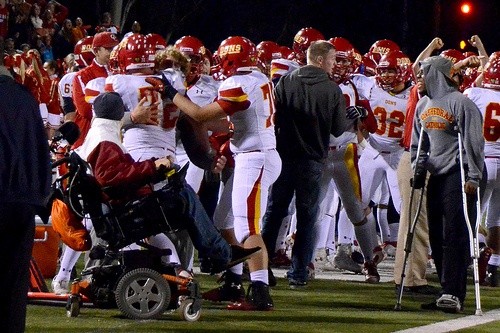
[74,36,95,66]
[108,27,500,90]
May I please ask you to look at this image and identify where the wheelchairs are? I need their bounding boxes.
[52,151,202,322]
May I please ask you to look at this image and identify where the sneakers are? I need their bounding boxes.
[313,245,364,274]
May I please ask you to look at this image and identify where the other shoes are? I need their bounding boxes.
[200,272,246,302]
[288,277,307,289]
[247,281,274,310]
[395,285,442,299]
[364,261,380,282]
[200,246,262,275]
[484,264,500,287]
[272,249,292,270]
[435,294,461,313]
[384,244,396,258]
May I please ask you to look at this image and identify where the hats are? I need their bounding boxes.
[94,92,124,120]
[92,32,119,48]
[66,53,75,64]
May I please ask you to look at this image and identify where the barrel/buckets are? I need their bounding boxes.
[30,215,61,278]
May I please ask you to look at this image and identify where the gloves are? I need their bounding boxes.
[346,105,367,119]
[145,73,178,101]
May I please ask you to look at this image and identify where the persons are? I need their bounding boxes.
[0,63,53,333]
[145,36,282,312]
[410,55,488,312]
[0,0,500,295]
